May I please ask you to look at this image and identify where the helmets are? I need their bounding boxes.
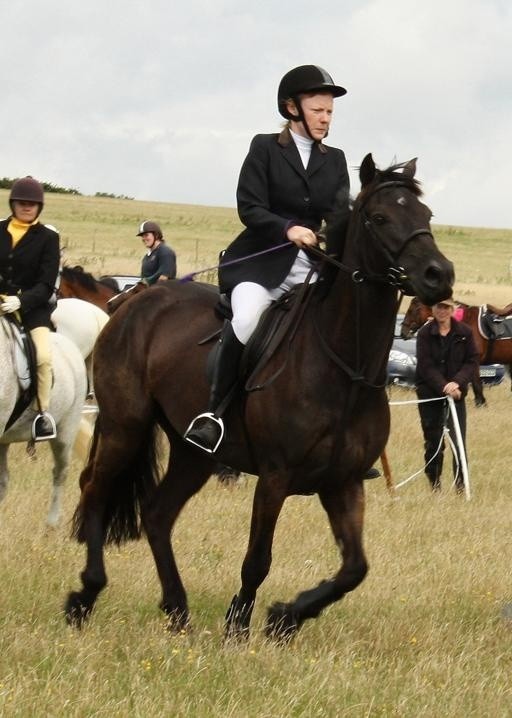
[135,221,160,236]
[278,65,347,121]
[10,176,44,205]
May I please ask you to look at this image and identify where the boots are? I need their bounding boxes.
[36,415,53,436]
[363,469,381,478]
[187,319,246,449]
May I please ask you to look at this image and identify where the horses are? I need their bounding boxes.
[60,149,456,643]
[400,295,512,409]
[0,263,152,534]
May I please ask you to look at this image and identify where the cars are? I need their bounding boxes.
[383,315,505,386]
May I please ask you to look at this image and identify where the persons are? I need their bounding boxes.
[0,176,60,436]
[136,220,176,286]
[416,297,478,494]
[188,65,349,460]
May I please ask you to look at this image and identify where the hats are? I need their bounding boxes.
[437,296,456,307]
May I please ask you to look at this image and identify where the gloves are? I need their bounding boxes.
[0,295,21,313]
